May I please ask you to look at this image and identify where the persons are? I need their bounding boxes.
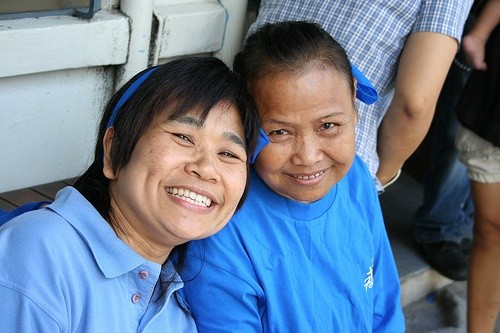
[446,0,500,333]
[239,0,476,200]
[170,20,411,333]
[399,59,476,282]
[0,54,261,333]
